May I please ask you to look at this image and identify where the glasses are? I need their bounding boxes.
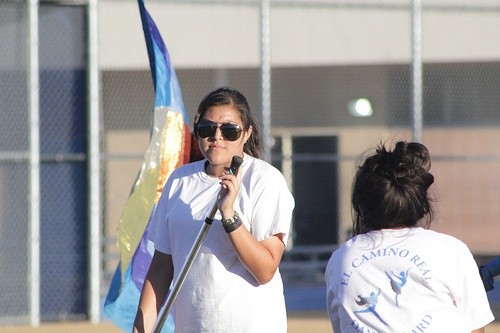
[197,120,250,141]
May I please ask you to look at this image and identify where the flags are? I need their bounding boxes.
[103,0,190,333]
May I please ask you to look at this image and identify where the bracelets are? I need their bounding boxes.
[221,211,239,225]
[224,218,242,234]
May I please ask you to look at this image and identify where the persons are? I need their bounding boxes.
[324,140,496,333]
[132,86,296,333]
